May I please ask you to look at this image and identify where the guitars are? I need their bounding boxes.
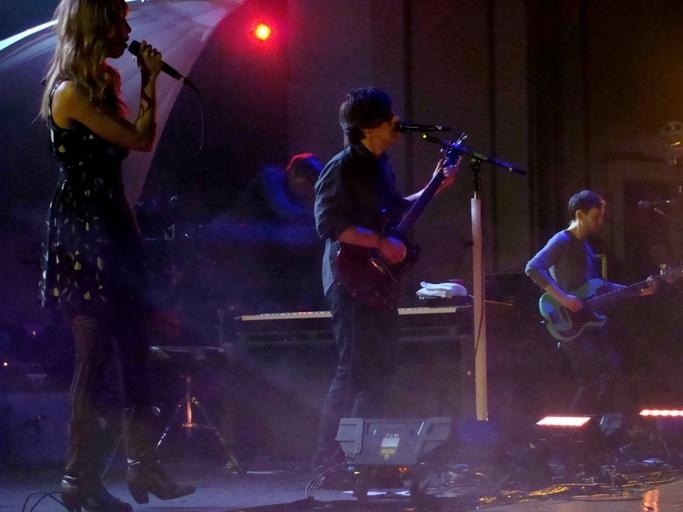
[538,268,683,342]
[335,130,470,311]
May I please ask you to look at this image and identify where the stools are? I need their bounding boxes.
[145,344,252,483]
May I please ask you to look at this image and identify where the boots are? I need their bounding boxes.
[59,417,134,511]
[122,406,196,504]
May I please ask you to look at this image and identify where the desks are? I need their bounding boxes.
[218,324,514,483]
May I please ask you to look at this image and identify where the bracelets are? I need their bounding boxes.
[375,234,383,250]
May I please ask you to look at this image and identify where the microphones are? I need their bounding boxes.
[636,200,674,226]
[395,120,448,133]
[127,39,183,80]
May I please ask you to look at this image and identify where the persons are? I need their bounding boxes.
[226,152,323,230]
[516,187,658,413]
[303,84,464,494]
[29,1,198,511]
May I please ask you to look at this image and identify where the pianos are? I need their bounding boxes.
[218,294,514,470]
